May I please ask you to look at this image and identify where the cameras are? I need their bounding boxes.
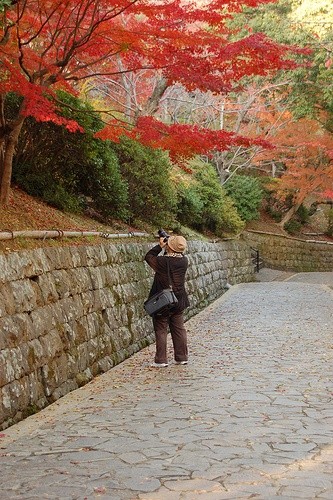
[158,228,171,245]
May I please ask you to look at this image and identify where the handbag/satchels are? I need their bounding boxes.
[143,287,179,317]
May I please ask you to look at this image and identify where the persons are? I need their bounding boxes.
[144,234,191,367]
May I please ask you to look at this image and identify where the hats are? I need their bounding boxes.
[168,235,187,252]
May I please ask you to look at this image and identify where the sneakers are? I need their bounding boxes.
[172,360,188,365]
[151,363,169,368]
